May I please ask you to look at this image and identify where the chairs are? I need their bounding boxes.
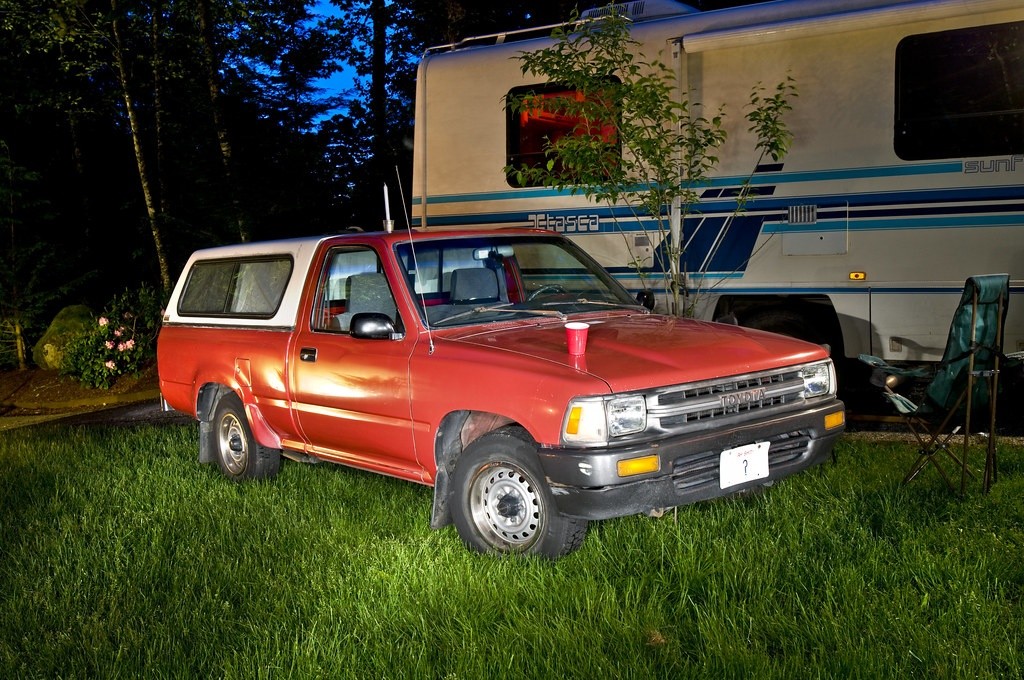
[332,266,517,341]
[859,272,1010,502]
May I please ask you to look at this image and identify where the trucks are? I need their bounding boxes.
[410,0,1024,401]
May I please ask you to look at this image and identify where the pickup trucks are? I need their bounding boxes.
[157,228,847,560]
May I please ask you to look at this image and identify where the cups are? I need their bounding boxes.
[567,354,587,375]
[564,322,590,356]
